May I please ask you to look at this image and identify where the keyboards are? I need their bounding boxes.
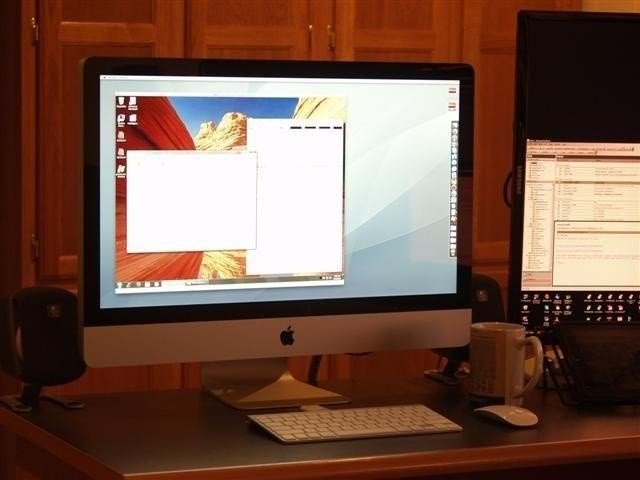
[247,404,463,444]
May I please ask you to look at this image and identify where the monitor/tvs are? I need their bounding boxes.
[507,10,640,408]
[79,57,475,409]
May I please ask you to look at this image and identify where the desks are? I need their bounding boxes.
[0,378,640,479]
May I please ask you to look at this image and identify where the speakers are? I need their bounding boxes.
[424,273,503,385]
[0,287,87,414]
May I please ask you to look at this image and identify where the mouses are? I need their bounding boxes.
[473,404,539,430]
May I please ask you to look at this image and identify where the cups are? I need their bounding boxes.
[470,323,544,410]
